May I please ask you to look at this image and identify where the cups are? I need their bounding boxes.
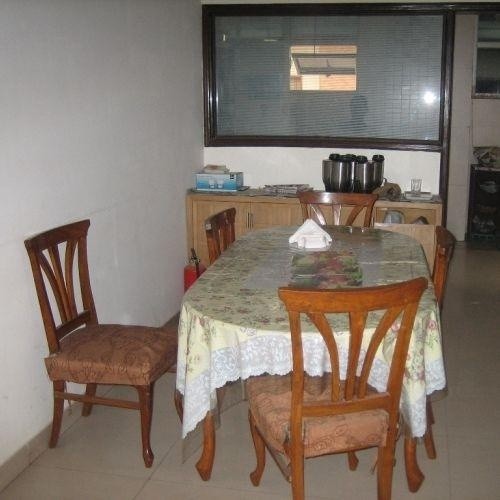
[411,179,423,198]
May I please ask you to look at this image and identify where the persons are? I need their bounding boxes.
[338,95,371,139]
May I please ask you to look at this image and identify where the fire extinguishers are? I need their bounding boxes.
[183,247,207,295]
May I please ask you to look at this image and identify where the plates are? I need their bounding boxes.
[404,191,433,201]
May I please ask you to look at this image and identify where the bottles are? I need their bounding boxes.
[321,153,383,192]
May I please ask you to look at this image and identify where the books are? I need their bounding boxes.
[400,189,440,204]
[194,163,313,197]
